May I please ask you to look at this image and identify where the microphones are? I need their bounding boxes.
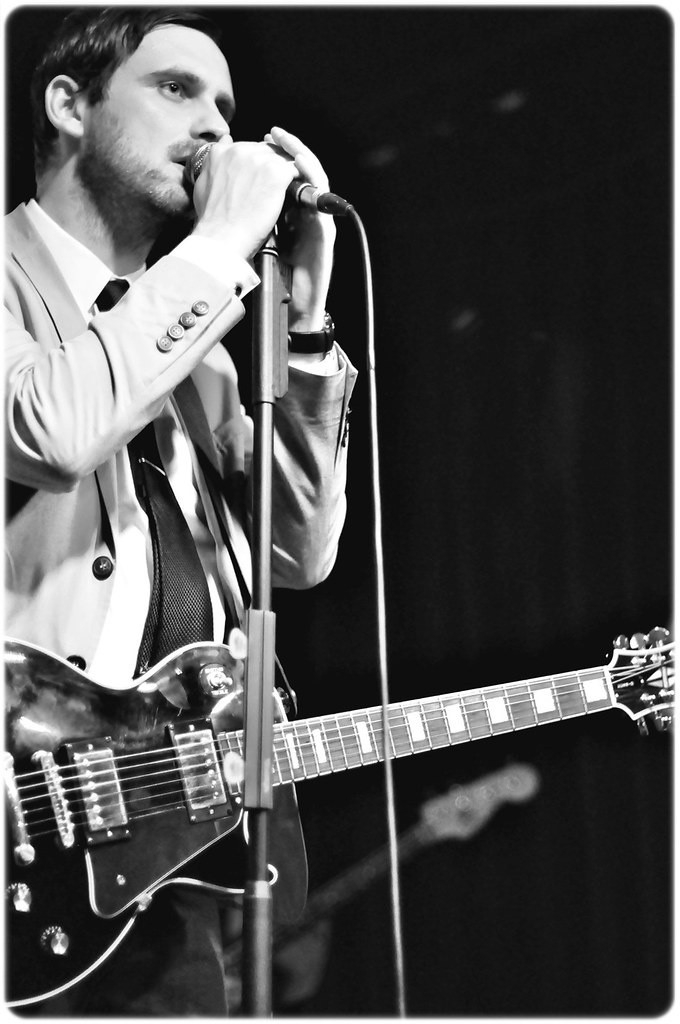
[186,142,354,217]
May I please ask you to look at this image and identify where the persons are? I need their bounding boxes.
[5,5,361,1018]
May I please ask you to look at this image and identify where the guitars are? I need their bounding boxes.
[3,626,675,1018]
[127,766,540,1015]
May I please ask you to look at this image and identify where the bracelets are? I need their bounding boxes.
[286,309,336,353]
